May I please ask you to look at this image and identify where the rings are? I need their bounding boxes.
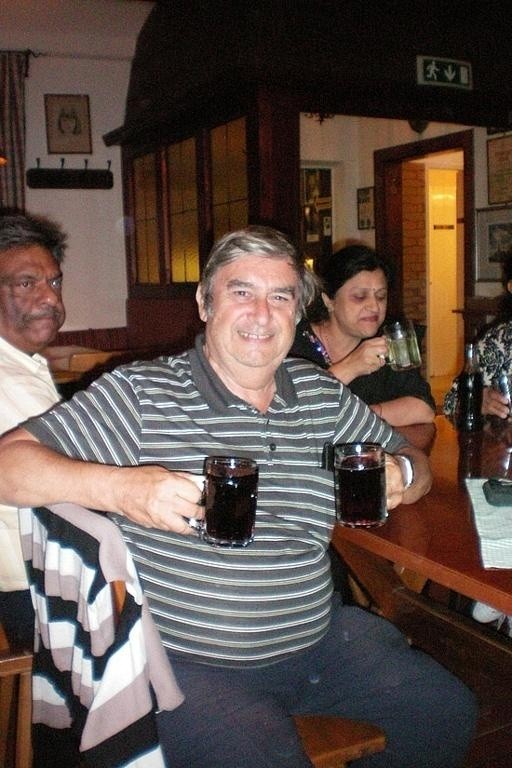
[378,354,386,359]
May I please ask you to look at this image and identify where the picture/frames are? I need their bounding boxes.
[486,134,512,205]
[44,94,93,154]
[357,186,375,230]
[474,205,512,282]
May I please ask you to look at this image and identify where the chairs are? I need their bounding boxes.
[0,648,34,768]
[69,348,150,372]
[111,581,386,768]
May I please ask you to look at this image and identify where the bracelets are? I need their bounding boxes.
[377,402,384,419]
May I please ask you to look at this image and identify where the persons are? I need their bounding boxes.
[0,225,476,767]
[443,254,511,441]
[49,109,84,152]
[286,243,437,425]
[1,208,66,673]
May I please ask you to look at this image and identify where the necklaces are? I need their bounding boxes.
[320,322,340,364]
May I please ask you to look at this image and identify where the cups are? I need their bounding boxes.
[491,363,511,407]
[327,440,388,531]
[183,458,258,547]
[490,445,512,481]
[380,320,422,374]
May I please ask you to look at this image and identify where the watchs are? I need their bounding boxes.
[394,453,415,490]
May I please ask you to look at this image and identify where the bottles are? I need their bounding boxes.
[452,433,488,524]
[456,338,486,434]
